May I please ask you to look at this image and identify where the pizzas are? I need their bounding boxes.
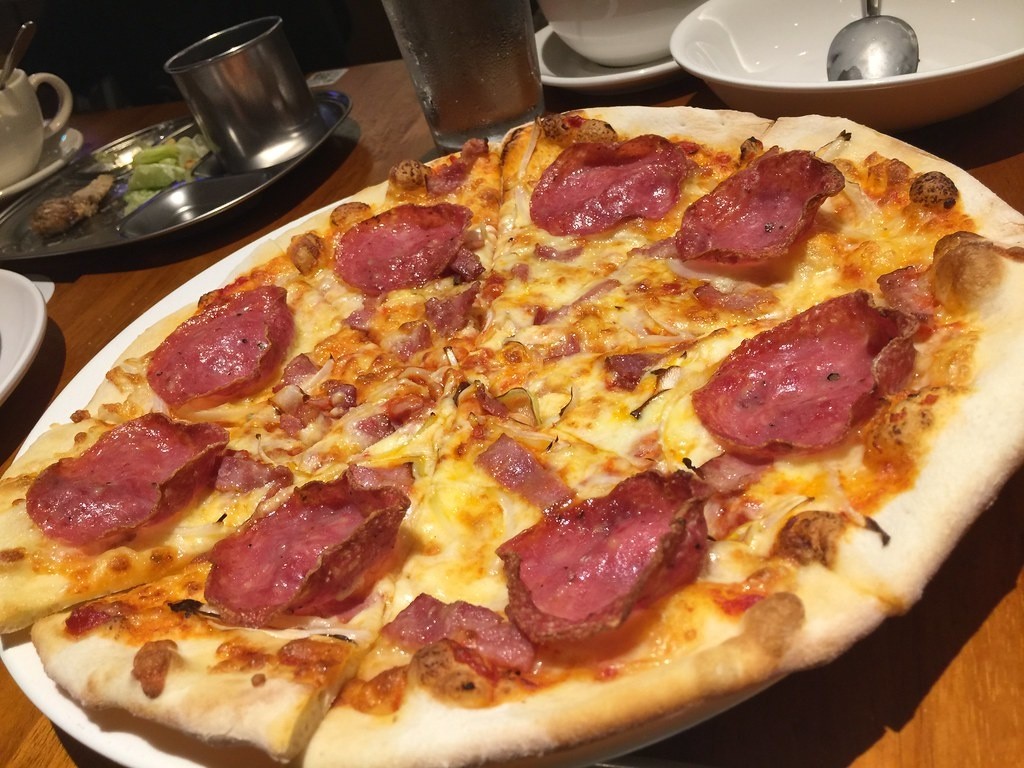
[0,106,1024,768]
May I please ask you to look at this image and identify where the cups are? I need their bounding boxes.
[165,15,324,173]
[381,0,547,159]
[0,69,74,190]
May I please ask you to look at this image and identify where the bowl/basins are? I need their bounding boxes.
[538,0,708,67]
[671,0,1024,132]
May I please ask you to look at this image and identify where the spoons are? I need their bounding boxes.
[826,0,919,82]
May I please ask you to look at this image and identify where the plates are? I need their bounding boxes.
[0,121,85,202]
[0,89,354,261]
[535,25,683,95]
[0,106,789,768]
[0,269,48,408]
